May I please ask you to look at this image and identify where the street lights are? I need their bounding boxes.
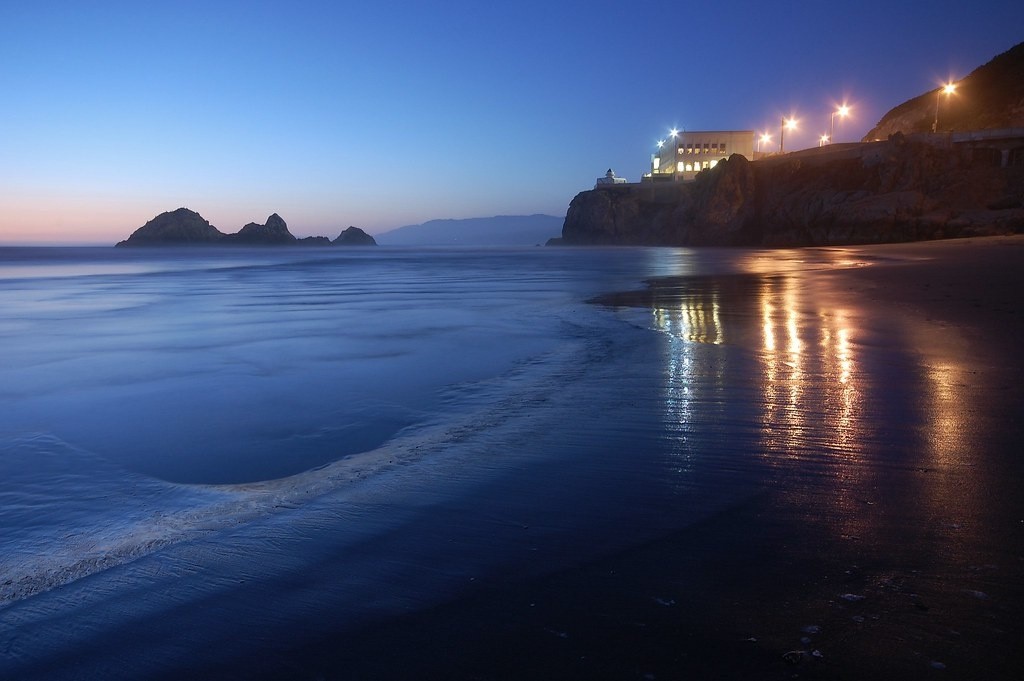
[658,140,663,159]
[781,116,795,153]
[819,135,827,148]
[831,106,847,145]
[757,132,768,153]
[933,84,953,133]
[672,130,678,176]
